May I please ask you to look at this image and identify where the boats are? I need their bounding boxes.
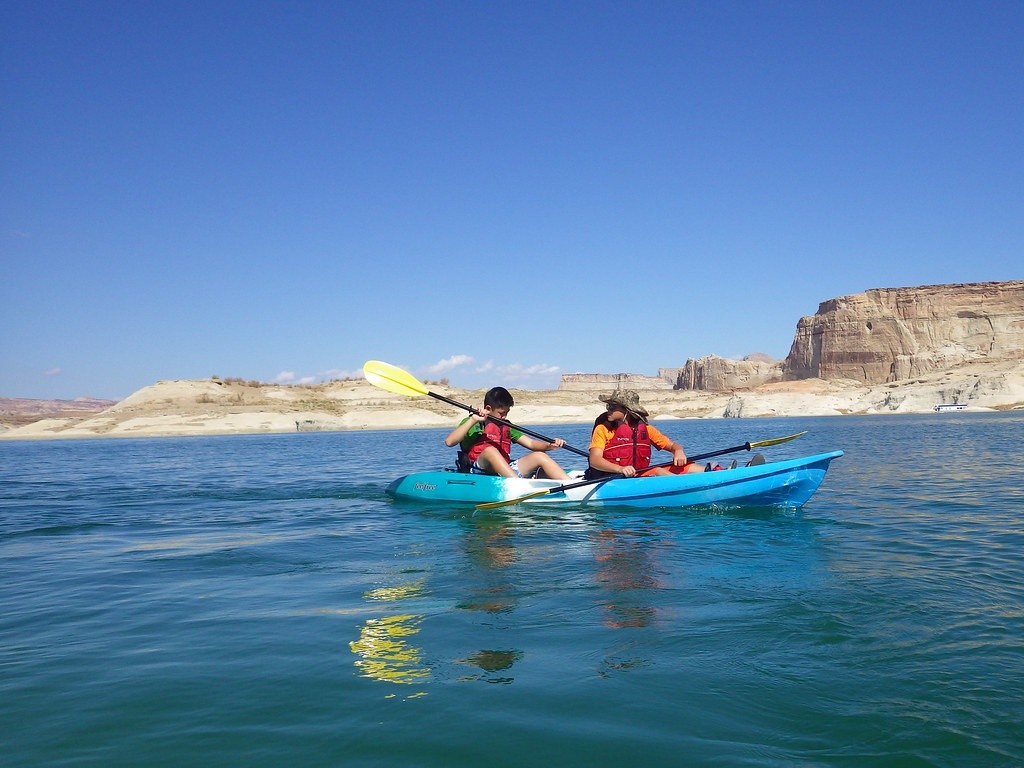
[384,450,845,508]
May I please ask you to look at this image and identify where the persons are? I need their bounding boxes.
[584,387,766,479]
[444,386,574,482]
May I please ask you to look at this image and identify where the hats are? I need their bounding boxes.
[598,387,649,420]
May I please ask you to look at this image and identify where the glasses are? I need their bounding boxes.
[606,403,622,411]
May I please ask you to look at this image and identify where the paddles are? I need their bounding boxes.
[362,359,592,459]
[473,431,812,510]
